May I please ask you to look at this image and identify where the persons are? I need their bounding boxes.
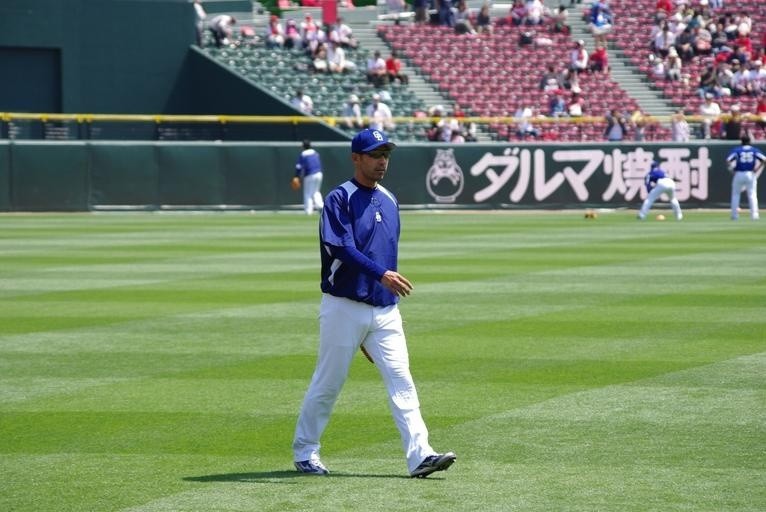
[342,92,394,132]
[725,135,766,223]
[290,91,312,115]
[637,161,683,222]
[292,140,325,216]
[604,108,648,141]
[387,0,617,51]
[648,1,766,144]
[426,102,476,143]
[267,1,358,73]
[292,129,456,479]
[208,16,237,49]
[515,40,607,134]
[193,0,207,49]
[366,51,408,87]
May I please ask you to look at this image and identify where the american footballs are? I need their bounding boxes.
[656,215,666,221]
[291,177,301,191]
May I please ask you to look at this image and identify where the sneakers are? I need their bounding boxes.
[293,458,330,478]
[409,451,456,479]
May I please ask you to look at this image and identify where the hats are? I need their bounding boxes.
[350,128,396,154]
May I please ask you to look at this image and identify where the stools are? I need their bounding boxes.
[190,0,765,149]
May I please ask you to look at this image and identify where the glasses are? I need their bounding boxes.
[358,150,391,159]
